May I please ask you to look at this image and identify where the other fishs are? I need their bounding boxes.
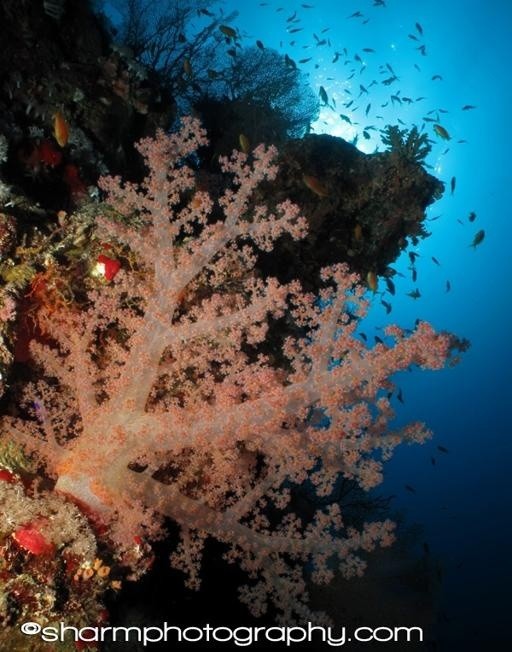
[112,0,489,652]
